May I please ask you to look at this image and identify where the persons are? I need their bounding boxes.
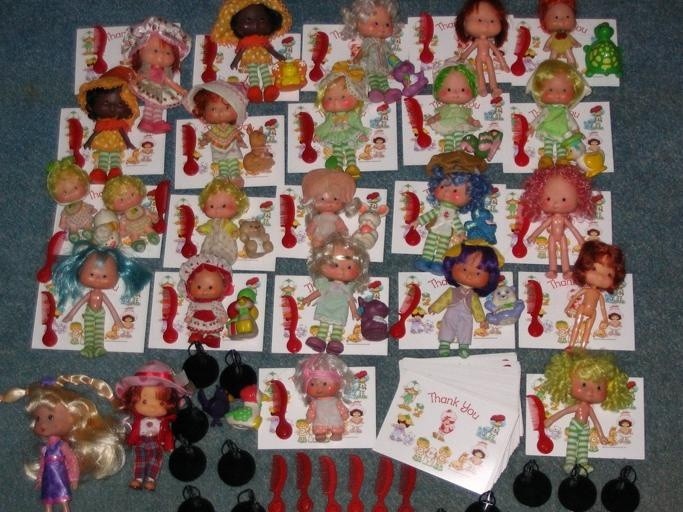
[0,0,630,511]
[1,0,631,512]
